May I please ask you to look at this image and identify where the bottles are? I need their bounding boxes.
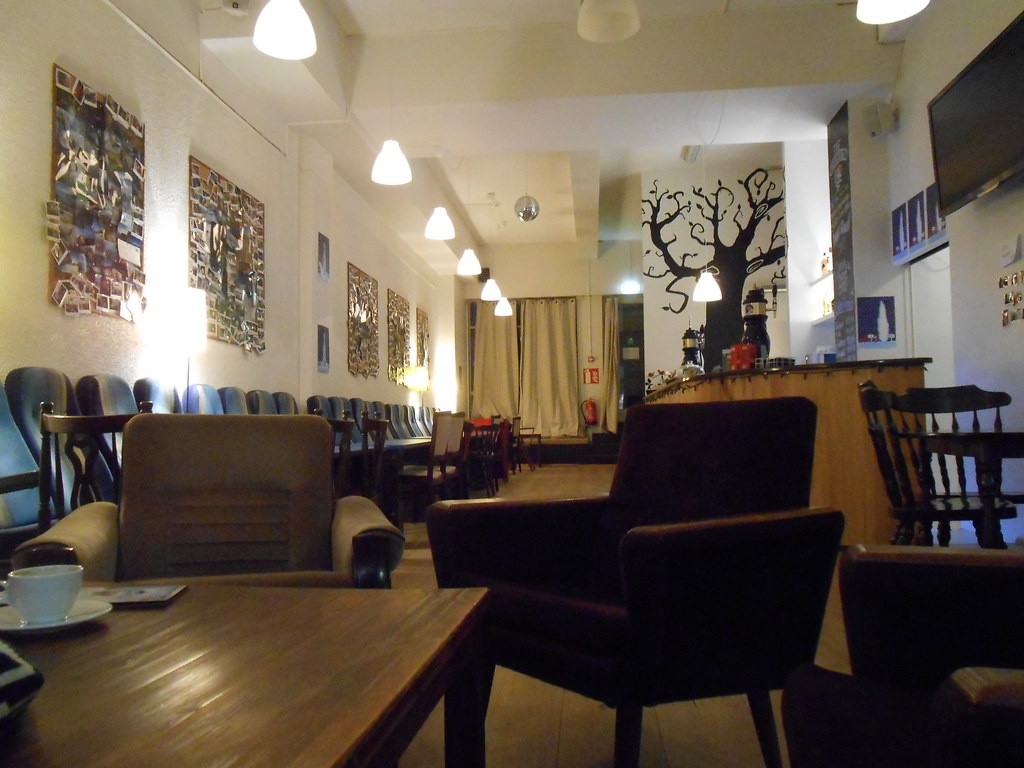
[722,350,732,373]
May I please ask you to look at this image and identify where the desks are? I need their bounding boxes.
[0,573,494,768]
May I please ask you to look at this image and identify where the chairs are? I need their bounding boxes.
[0,365,1024,768]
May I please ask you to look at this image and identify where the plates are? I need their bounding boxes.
[0,599,116,636]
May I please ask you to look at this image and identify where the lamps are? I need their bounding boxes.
[424,206,455,240]
[856,0,930,26]
[693,145,722,302]
[456,249,513,317]
[251,0,317,61]
[576,0,641,44]
[371,136,413,186]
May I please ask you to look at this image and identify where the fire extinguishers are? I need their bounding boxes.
[581,398,598,425]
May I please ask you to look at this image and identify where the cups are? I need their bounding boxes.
[0,565,85,624]
[825,353,837,363]
